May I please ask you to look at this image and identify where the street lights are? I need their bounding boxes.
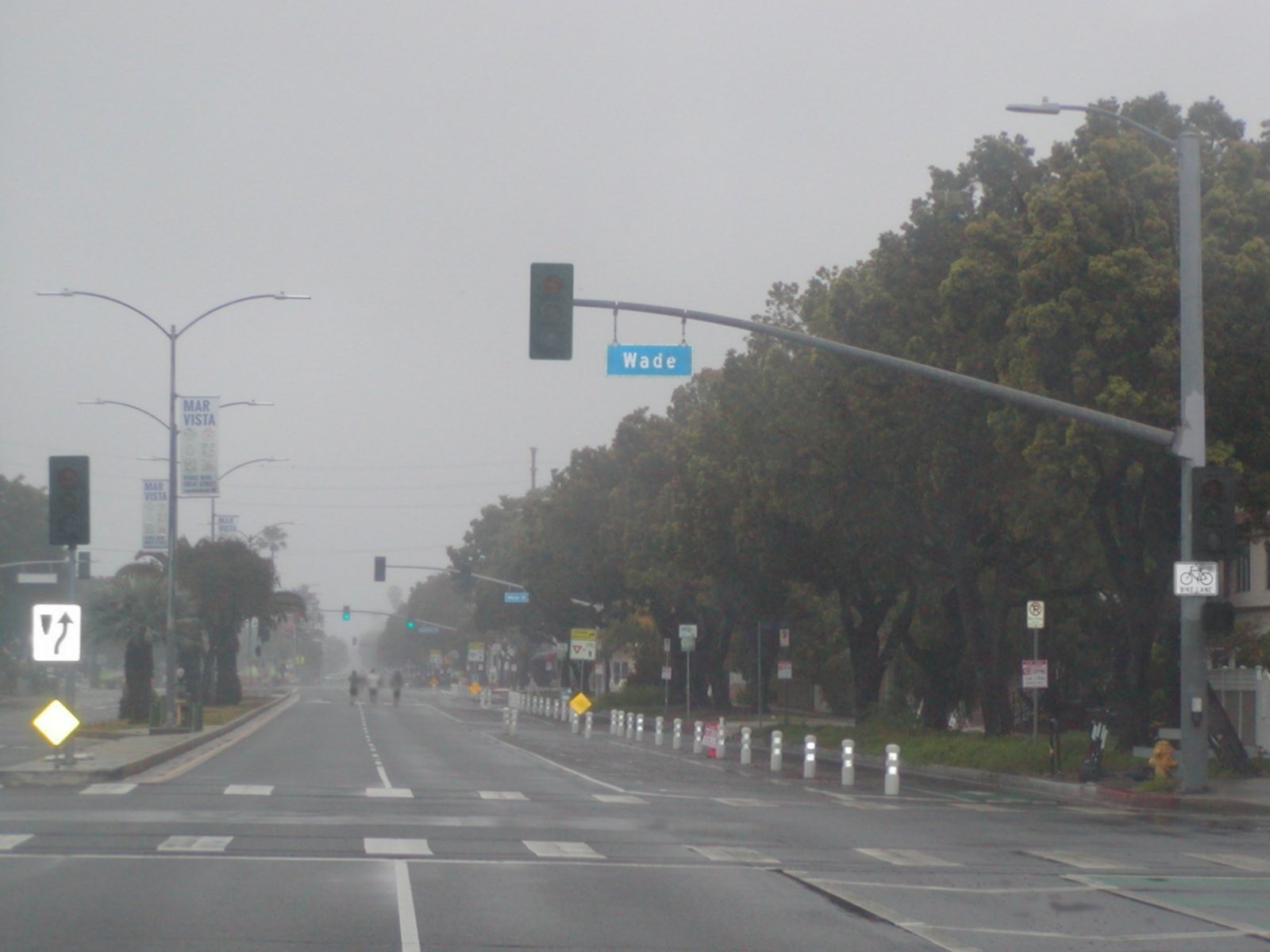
[82,396,287,687]
[37,286,315,728]
[1009,100,1217,795]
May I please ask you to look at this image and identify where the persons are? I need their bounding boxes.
[368,671,380,706]
[1090,715,1108,776]
[348,669,363,705]
[389,671,405,707]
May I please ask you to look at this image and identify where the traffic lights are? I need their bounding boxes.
[529,262,574,359]
[343,606,350,619]
[407,614,414,628]
[374,556,386,581]
[50,455,92,545]
